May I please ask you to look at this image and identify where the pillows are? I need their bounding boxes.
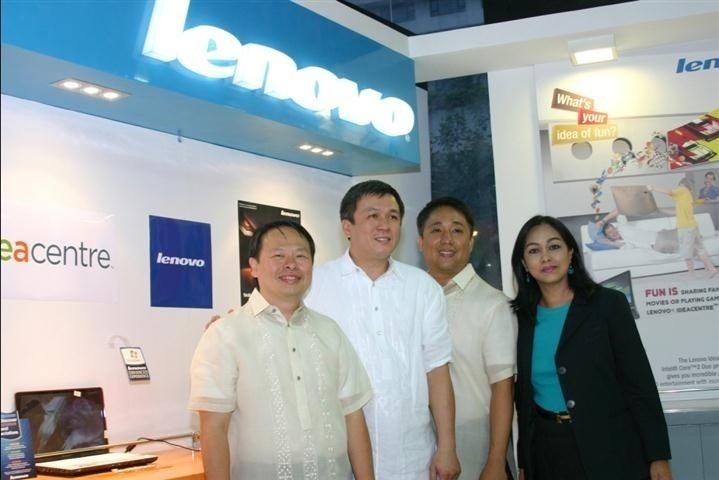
[587,219,607,241]
[651,227,683,254]
[610,183,661,219]
[584,241,614,251]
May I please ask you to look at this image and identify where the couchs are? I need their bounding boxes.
[578,210,719,283]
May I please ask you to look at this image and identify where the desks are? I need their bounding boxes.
[18,435,206,480]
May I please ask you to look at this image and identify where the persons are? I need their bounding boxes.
[696,171,719,204]
[204,180,461,480]
[643,175,719,280]
[594,206,719,251]
[187,218,378,480]
[415,195,521,479]
[507,214,673,480]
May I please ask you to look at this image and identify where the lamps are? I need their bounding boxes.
[566,32,619,69]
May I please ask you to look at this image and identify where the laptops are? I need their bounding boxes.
[15,387,159,475]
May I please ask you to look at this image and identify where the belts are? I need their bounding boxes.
[531,411,573,423]
